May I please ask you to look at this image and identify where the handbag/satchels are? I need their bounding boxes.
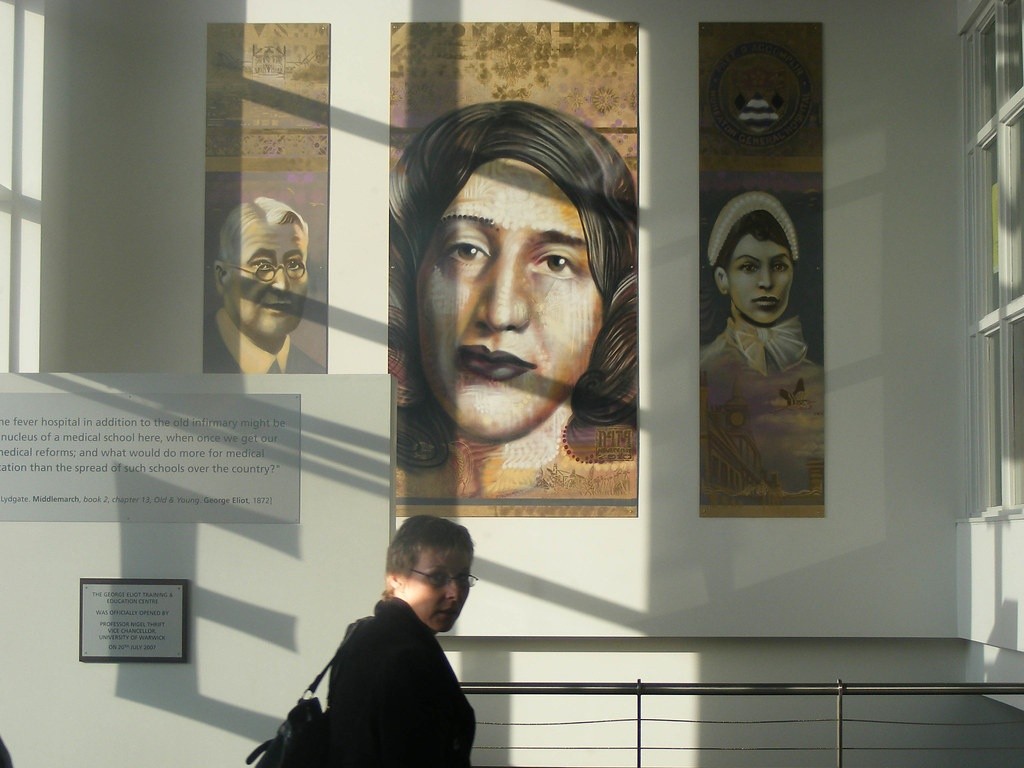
[246,616,373,768]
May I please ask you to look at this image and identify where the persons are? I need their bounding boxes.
[322,515,476,768]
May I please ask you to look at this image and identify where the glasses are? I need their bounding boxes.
[411,568,478,587]
